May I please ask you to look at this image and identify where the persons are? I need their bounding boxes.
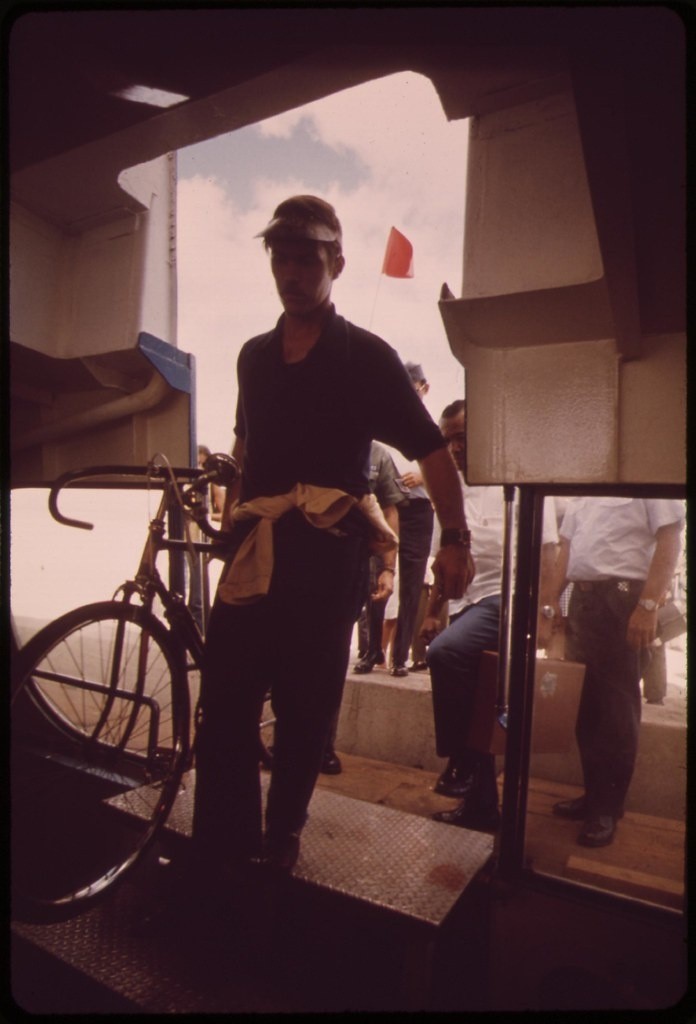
[426,399,559,832]
[135,196,467,933]
[354,362,438,677]
[549,496,686,847]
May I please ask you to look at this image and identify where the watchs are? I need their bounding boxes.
[541,606,556,618]
[441,528,470,549]
[637,599,660,612]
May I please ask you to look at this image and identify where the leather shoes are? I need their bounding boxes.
[435,760,470,796]
[433,802,502,832]
[553,796,604,818]
[577,813,617,848]
[320,750,341,776]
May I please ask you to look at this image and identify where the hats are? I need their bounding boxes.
[263,219,339,242]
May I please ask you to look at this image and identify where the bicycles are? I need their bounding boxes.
[2,455,282,929]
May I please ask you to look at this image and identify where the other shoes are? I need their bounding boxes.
[355,648,429,677]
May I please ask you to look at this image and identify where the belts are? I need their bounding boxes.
[577,577,647,597]
